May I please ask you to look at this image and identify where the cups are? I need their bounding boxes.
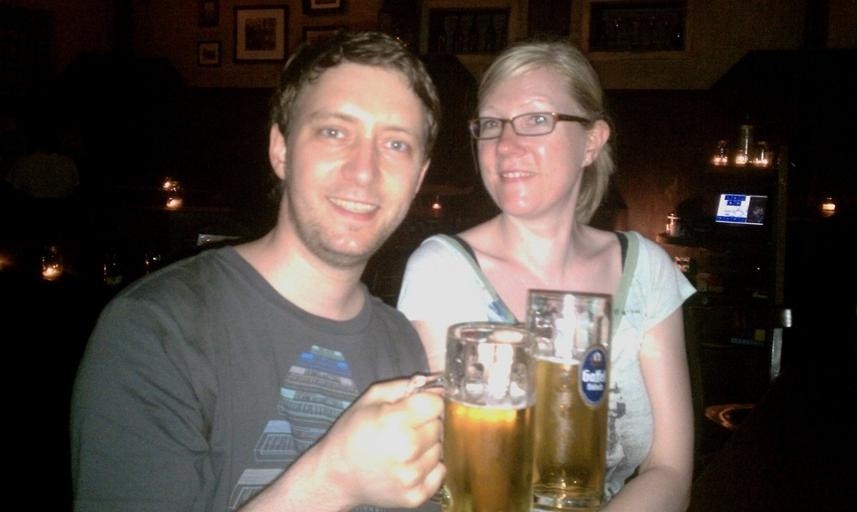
[420,322,539,511]
[526,288,612,512]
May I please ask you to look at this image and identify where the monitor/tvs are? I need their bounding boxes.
[715,192,767,226]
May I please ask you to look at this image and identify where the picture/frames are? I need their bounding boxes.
[302,0,344,15]
[233,5,288,63]
[198,0,219,26]
[303,26,348,46]
[197,41,222,67]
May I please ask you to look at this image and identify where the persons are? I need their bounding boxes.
[395,39,701,512]
[67,29,448,512]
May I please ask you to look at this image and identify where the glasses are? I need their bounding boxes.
[469,112,590,140]
